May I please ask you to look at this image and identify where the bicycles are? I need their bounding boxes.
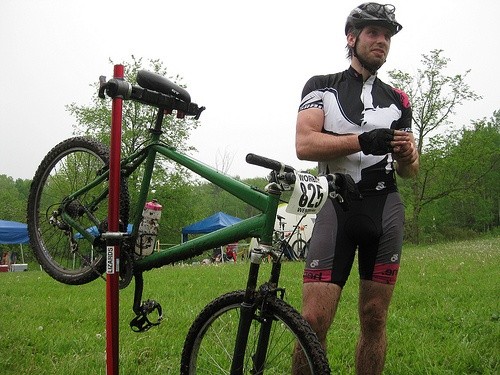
[27,64,360,375]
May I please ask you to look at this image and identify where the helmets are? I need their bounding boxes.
[345,2,403,36]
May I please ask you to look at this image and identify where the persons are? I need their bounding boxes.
[292,3,419,375]
[1,250,18,265]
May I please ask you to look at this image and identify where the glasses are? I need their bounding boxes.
[361,2,396,14]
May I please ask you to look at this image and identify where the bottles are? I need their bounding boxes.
[135,198,162,256]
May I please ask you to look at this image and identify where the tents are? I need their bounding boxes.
[0,220,30,265]
[72,222,134,270]
[248,203,316,258]
[182,212,246,264]
